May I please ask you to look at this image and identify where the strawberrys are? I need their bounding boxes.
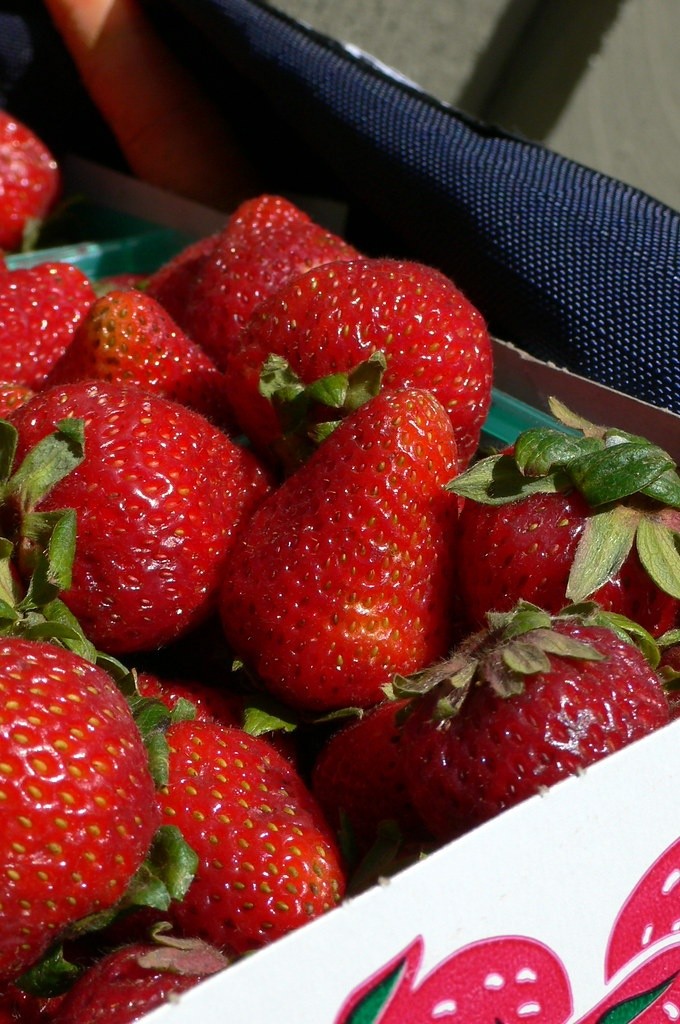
[0,110,680,1024]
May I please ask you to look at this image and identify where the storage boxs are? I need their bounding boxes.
[1,157,678,1024]
